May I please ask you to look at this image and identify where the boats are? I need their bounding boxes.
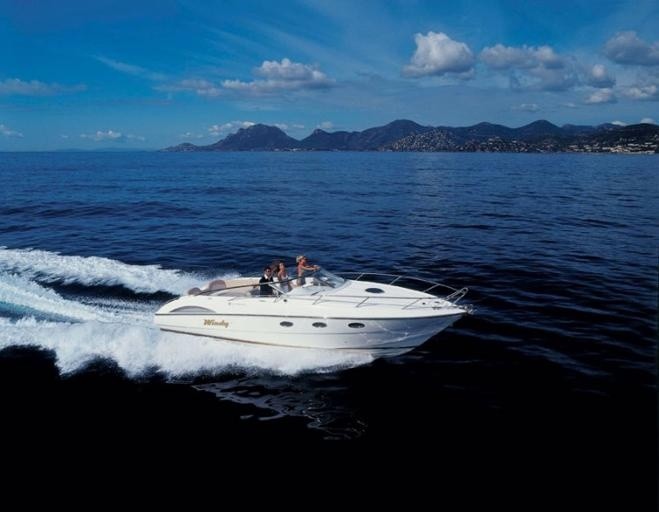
[154,271,474,358]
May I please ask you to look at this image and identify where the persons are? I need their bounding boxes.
[296,255,320,286]
[259,266,274,296]
[271,261,295,290]
